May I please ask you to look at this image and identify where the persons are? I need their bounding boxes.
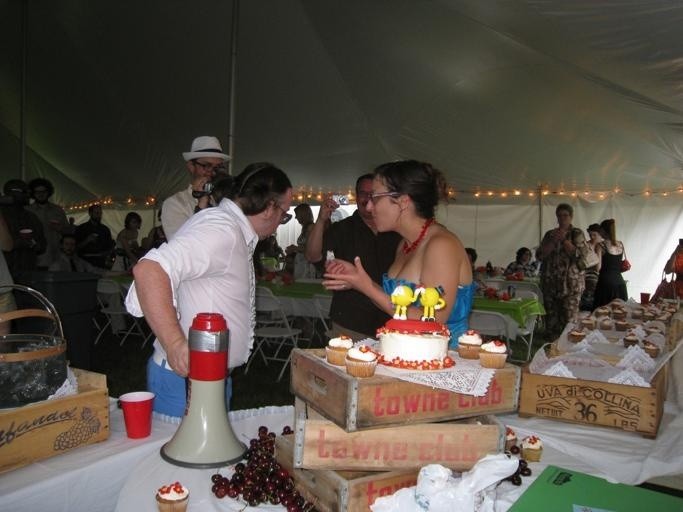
[590,218,628,314]
[122,160,294,425]
[664,238,683,299]
[316,157,473,360]
[461,247,478,270]
[300,170,406,342]
[534,202,590,342]
[503,247,536,277]
[159,134,237,243]
[577,222,607,316]
[2,176,319,401]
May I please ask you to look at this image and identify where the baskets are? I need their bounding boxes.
[0,284,67,409]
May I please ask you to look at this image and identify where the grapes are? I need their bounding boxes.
[511,446,520,454]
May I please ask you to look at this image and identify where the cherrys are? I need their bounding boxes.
[497,460,531,486]
[211,426,317,512]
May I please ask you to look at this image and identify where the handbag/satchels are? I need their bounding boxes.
[651,278,676,303]
[59,254,85,272]
[620,258,631,274]
[578,251,600,270]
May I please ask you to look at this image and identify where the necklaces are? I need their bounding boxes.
[401,215,436,257]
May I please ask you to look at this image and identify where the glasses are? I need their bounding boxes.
[8,187,26,193]
[278,205,292,224]
[368,192,401,202]
[93,211,103,215]
[35,190,47,194]
[196,163,221,171]
[557,212,570,217]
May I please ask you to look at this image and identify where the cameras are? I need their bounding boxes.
[332,194,349,205]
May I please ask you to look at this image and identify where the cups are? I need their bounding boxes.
[640,292,650,304]
[119,391,154,440]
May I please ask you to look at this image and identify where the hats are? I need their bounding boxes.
[182,136,233,162]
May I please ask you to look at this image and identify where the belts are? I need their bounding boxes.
[152,351,172,372]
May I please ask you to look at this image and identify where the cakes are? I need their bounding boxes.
[581,316,597,330]
[645,327,663,335]
[505,428,517,450]
[595,302,628,318]
[326,335,353,366]
[645,342,659,358]
[376,319,456,370]
[479,340,508,369]
[155,482,190,512]
[631,302,678,325]
[568,328,586,343]
[615,319,629,331]
[521,435,543,462]
[623,333,639,348]
[458,330,482,359]
[600,318,613,330]
[344,346,379,378]
